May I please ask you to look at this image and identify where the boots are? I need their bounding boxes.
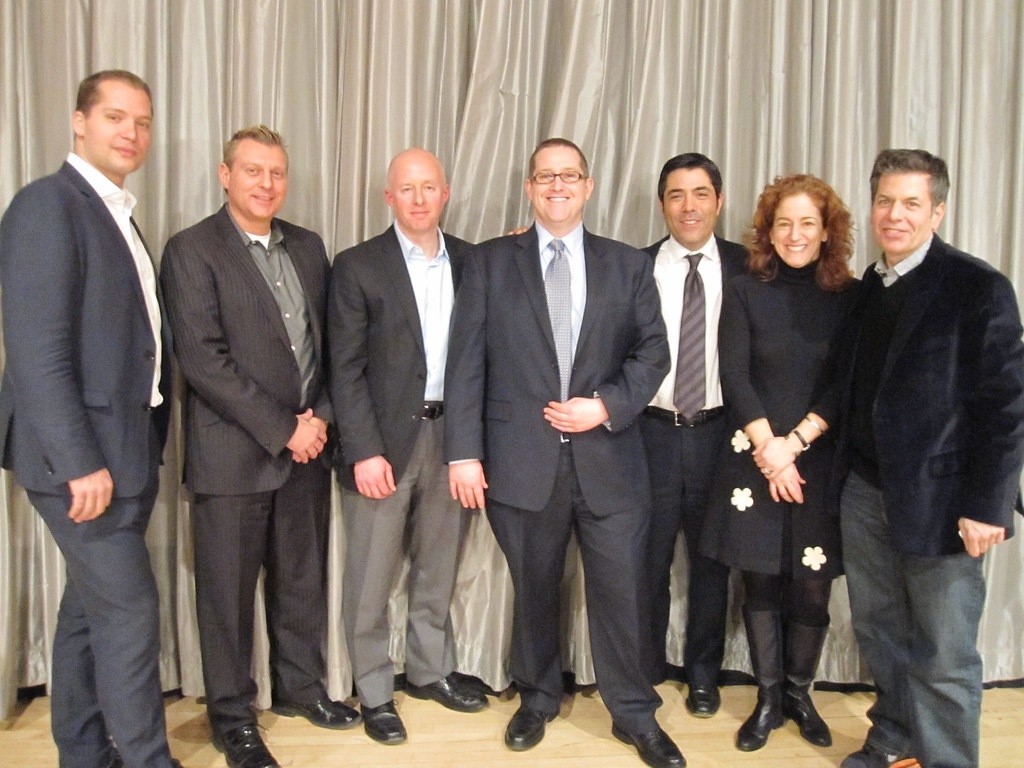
[735,604,790,751]
[784,612,832,748]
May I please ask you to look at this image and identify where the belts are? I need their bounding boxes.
[643,405,724,429]
[560,431,570,443]
[423,404,442,420]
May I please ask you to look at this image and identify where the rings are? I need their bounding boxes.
[764,468,771,474]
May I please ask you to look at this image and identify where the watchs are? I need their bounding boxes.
[792,429,810,451]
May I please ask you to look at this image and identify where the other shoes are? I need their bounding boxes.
[840,743,920,768]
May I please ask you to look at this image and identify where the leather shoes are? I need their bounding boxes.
[611,721,687,767]
[405,674,489,711]
[686,679,720,717]
[651,670,669,686]
[505,703,560,751]
[271,692,362,729]
[360,698,407,743]
[212,724,279,768]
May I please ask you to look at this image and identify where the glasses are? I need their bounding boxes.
[532,170,588,184]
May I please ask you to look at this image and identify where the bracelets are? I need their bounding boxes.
[803,416,826,435]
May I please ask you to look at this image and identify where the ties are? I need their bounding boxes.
[672,253,706,419]
[544,238,572,404]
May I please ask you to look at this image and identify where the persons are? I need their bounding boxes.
[327,149,486,746]
[0,69,185,768]
[629,153,754,717]
[157,127,361,768]
[696,172,863,752]
[441,137,687,768]
[837,146,1024,768]
